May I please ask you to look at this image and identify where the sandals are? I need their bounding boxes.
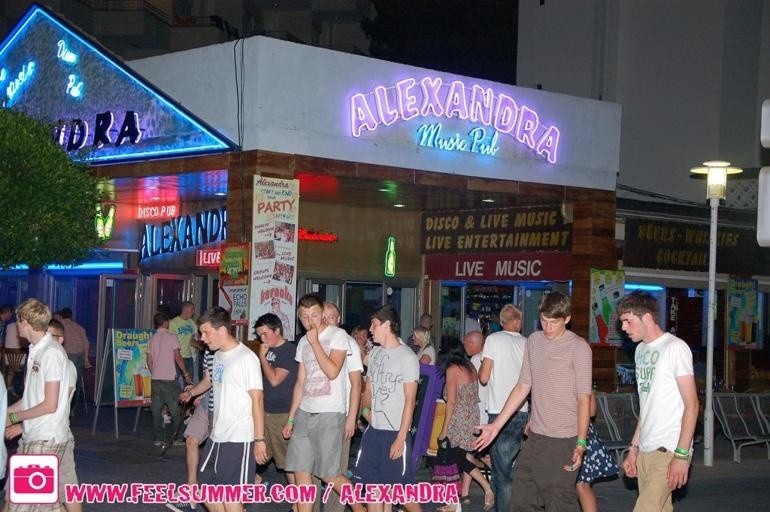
[484,489,494,512]
[436,495,472,512]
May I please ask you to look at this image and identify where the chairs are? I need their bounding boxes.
[1,346,29,404]
[67,350,91,419]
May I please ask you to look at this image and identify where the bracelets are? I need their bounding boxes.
[627,440,638,451]
[672,446,690,460]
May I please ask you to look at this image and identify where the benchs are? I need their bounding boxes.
[591,391,770,471]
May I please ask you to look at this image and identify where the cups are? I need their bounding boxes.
[132,372,144,395]
[143,376,153,397]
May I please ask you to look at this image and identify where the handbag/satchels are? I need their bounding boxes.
[432,436,462,497]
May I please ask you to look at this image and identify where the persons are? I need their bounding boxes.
[143,288,620,511]
[615,287,700,512]
[0,297,93,512]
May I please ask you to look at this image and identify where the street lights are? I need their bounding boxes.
[689,159,745,467]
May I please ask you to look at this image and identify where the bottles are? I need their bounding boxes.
[612,291,621,316]
[591,302,610,344]
[598,283,614,324]
